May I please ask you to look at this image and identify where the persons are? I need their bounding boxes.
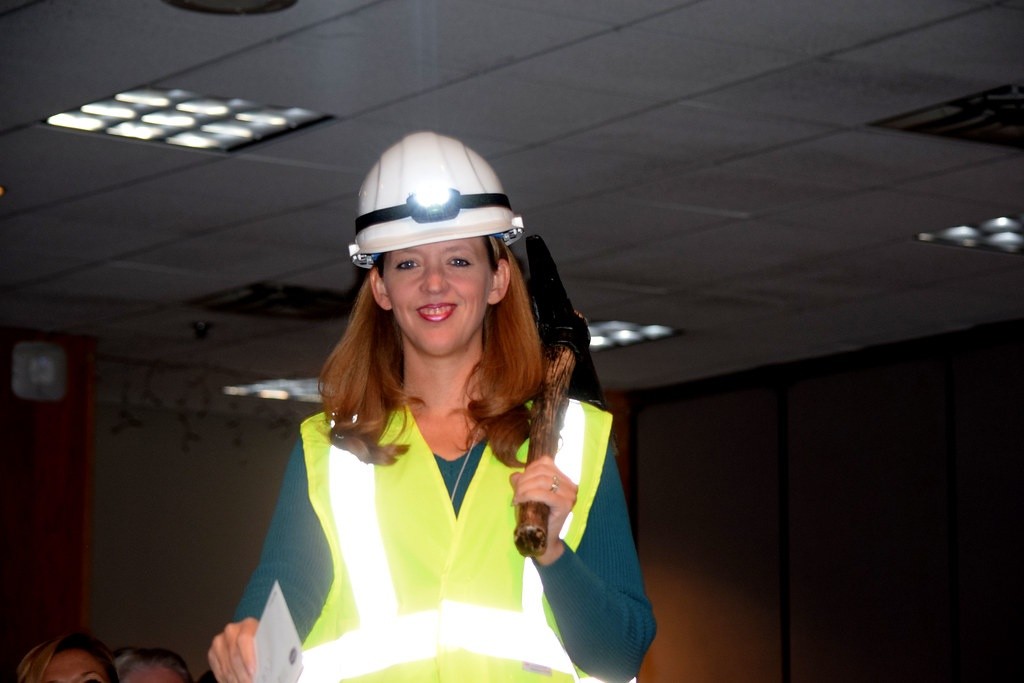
[208,133,658,683]
[17,633,120,683]
[110,646,195,683]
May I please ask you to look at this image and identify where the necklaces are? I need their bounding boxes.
[449,423,483,510]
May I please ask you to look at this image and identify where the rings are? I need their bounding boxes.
[550,476,561,492]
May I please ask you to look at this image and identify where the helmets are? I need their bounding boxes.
[349,131,525,270]
